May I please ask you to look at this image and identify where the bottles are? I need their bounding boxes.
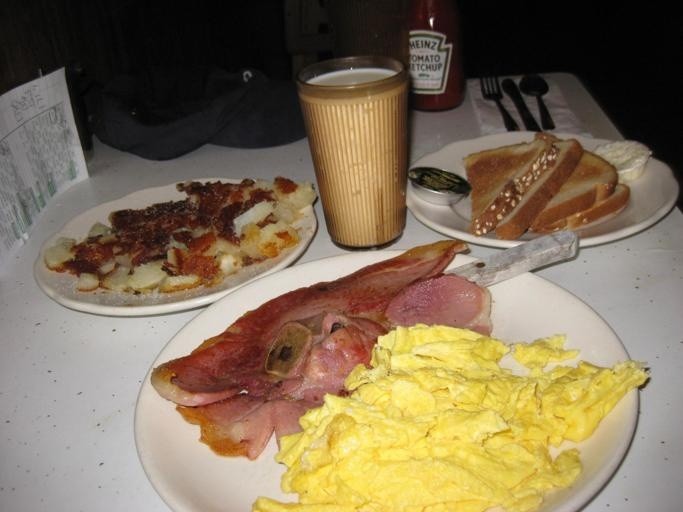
[405,0,468,116]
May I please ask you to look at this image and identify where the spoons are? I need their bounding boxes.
[518,71,556,131]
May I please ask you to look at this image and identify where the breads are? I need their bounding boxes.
[464,127,631,240]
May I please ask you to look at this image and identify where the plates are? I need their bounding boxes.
[31,174,321,321]
[404,130,681,251]
[130,248,635,509]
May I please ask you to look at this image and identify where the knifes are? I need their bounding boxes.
[501,75,541,131]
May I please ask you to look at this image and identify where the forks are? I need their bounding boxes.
[479,73,519,132]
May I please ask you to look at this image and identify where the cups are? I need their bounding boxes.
[292,54,414,251]
